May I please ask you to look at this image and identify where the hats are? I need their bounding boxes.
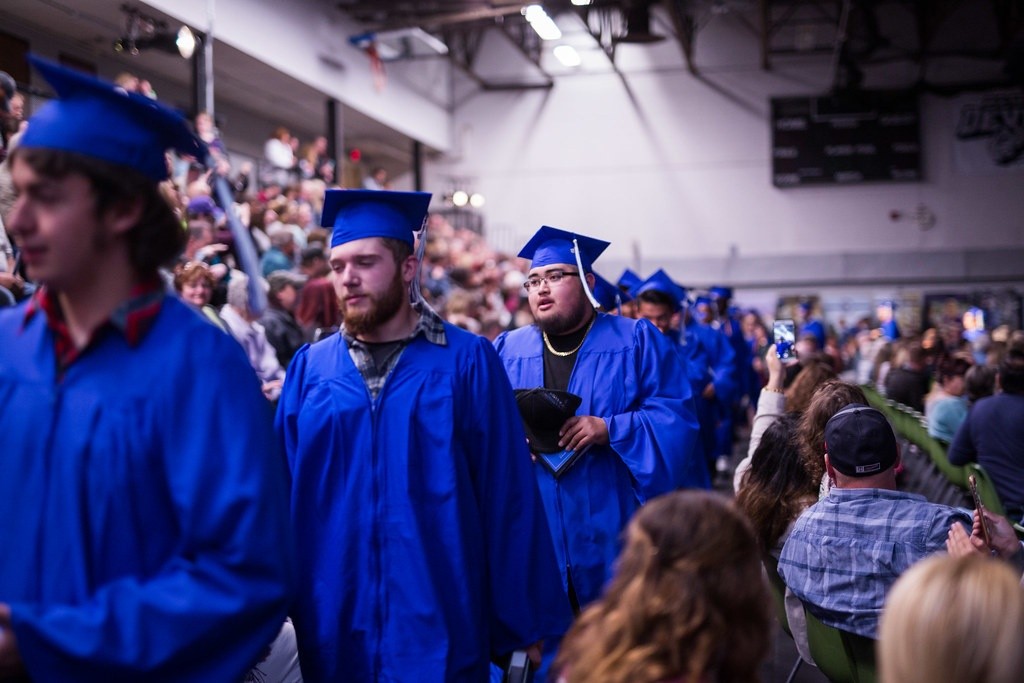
[18,51,267,313]
[636,270,687,346]
[307,228,328,250]
[822,404,899,475]
[321,188,439,319]
[300,241,327,261]
[694,285,732,304]
[260,247,292,277]
[801,321,825,348]
[268,271,307,290]
[877,300,895,309]
[616,270,643,295]
[188,196,223,219]
[799,303,812,312]
[517,226,611,310]
[591,271,632,317]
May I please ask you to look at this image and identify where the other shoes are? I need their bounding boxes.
[717,467,729,479]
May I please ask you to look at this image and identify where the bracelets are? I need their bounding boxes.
[761,386,785,394]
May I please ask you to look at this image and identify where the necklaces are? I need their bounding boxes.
[542,320,594,356]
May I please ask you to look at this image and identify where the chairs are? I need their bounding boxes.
[784,597,882,682]
[856,379,1024,556]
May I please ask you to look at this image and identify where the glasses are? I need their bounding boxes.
[523,272,581,293]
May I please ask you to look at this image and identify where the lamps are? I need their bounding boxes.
[115,9,196,59]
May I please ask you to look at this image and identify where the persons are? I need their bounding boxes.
[0,53,279,683]
[0,66,537,408]
[879,551,1024,683]
[945,505,1024,595]
[553,490,777,683]
[589,267,1024,595]
[493,226,712,683]
[273,190,569,683]
[778,402,995,683]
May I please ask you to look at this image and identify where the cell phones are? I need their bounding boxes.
[968,474,992,545]
[773,319,796,362]
